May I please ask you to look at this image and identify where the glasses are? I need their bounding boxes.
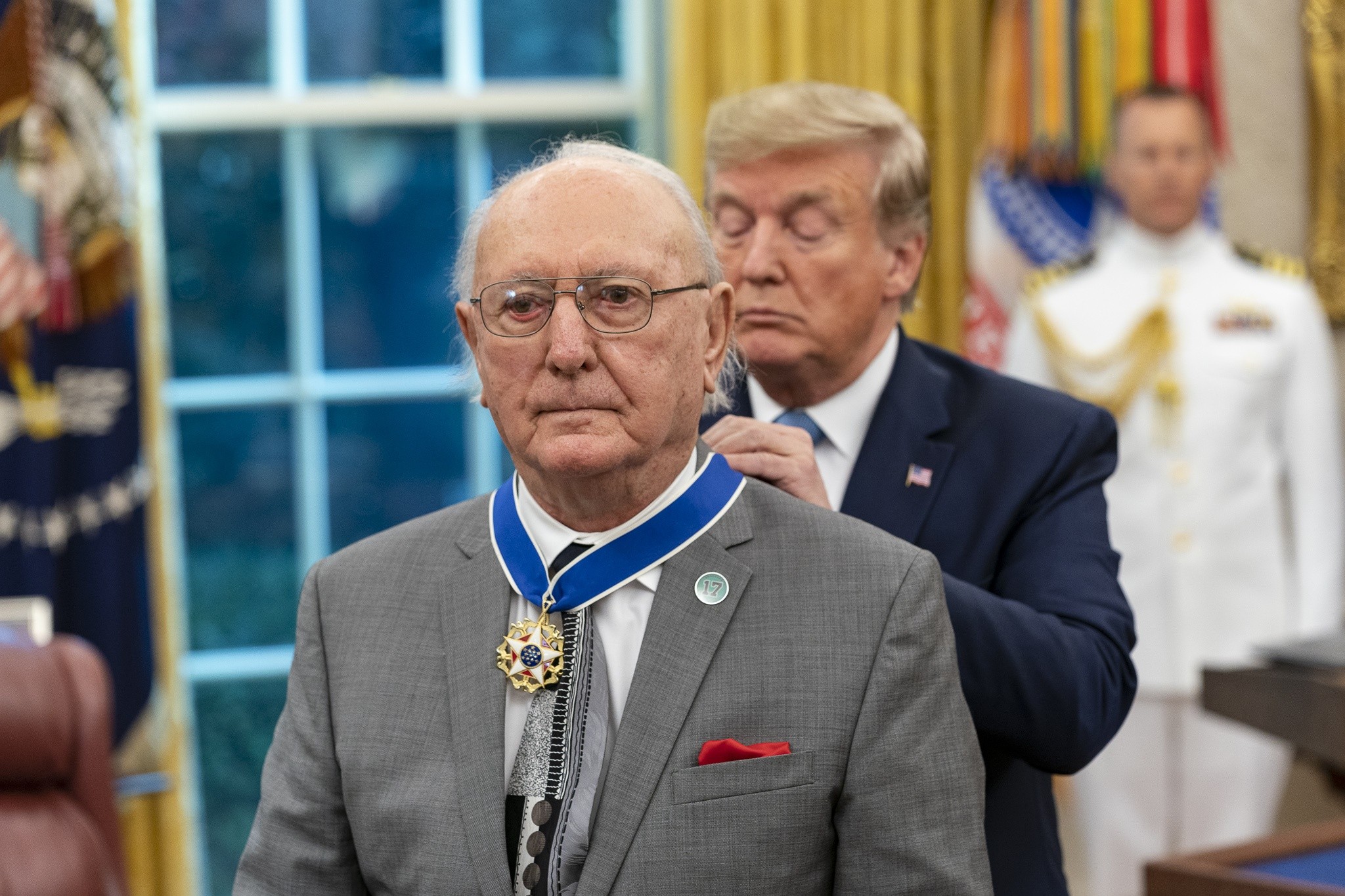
[469,274,708,338]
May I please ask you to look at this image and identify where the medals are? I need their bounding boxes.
[498,612,566,694]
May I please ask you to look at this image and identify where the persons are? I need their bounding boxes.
[0,595,127,896]
[231,138,995,896]
[998,88,1345,665]
[703,86,1138,896]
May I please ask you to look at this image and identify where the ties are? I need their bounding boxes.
[772,411,824,448]
[505,541,615,896]
